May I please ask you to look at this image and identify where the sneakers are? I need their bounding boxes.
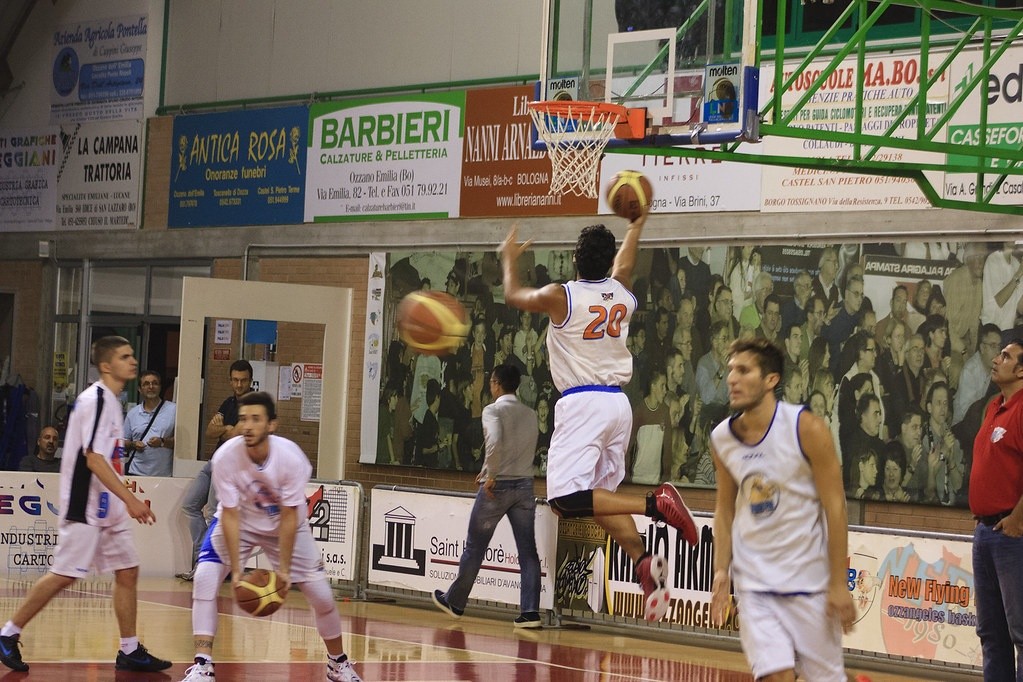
[182,657,216,682]
[0,633,29,672]
[513,612,542,628]
[633,554,671,623]
[432,588,464,619]
[652,480,699,547]
[116,642,172,672]
[175,569,196,581]
[325,654,363,682]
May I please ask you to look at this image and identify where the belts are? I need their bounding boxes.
[974,511,1013,524]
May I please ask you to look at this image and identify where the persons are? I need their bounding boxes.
[123,370,177,476]
[176,389,368,682]
[174,359,255,581]
[968,334,1023,681]
[493,203,698,627]
[708,335,856,682]
[20,424,62,473]
[0,335,171,672]
[379,238,1023,509]
[431,354,541,627]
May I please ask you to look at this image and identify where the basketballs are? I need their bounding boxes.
[605,170,653,218]
[709,78,736,103]
[234,569,287,617]
[551,90,572,101]
[395,289,472,355]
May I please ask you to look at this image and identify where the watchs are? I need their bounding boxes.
[159,438,164,447]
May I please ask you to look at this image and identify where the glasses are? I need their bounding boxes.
[489,380,501,386]
[826,259,840,265]
[679,340,694,348]
[865,346,877,355]
[813,309,827,318]
[850,289,866,300]
[636,331,646,339]
[718,299,734,305]
[798,283,815,290]
[984,341,1005,350]
[714,334,730,342]
[905,345,927,358]
[140,379,159,389]
